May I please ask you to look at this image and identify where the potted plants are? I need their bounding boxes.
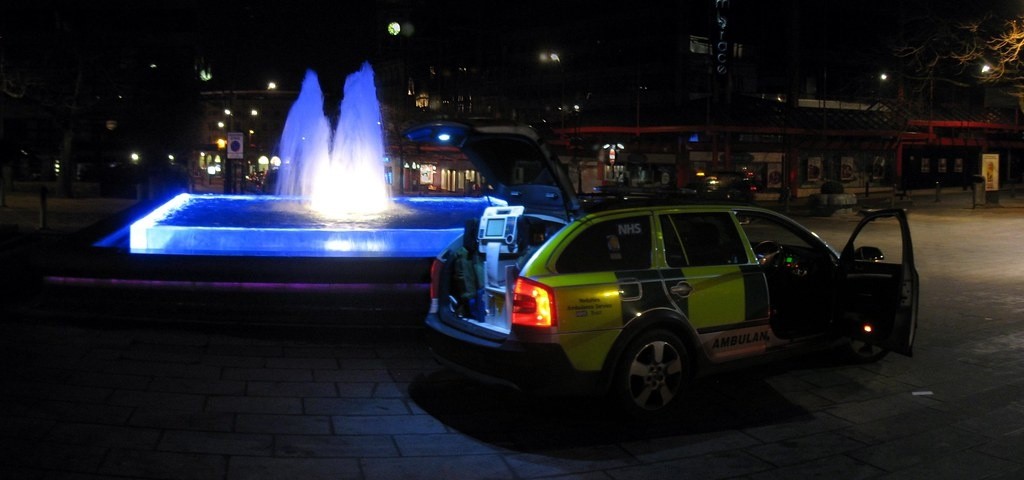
[808,181,858,215]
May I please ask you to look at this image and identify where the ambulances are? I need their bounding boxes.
[399,118,923,442]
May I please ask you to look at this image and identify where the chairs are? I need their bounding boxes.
[687,223,726,266]
